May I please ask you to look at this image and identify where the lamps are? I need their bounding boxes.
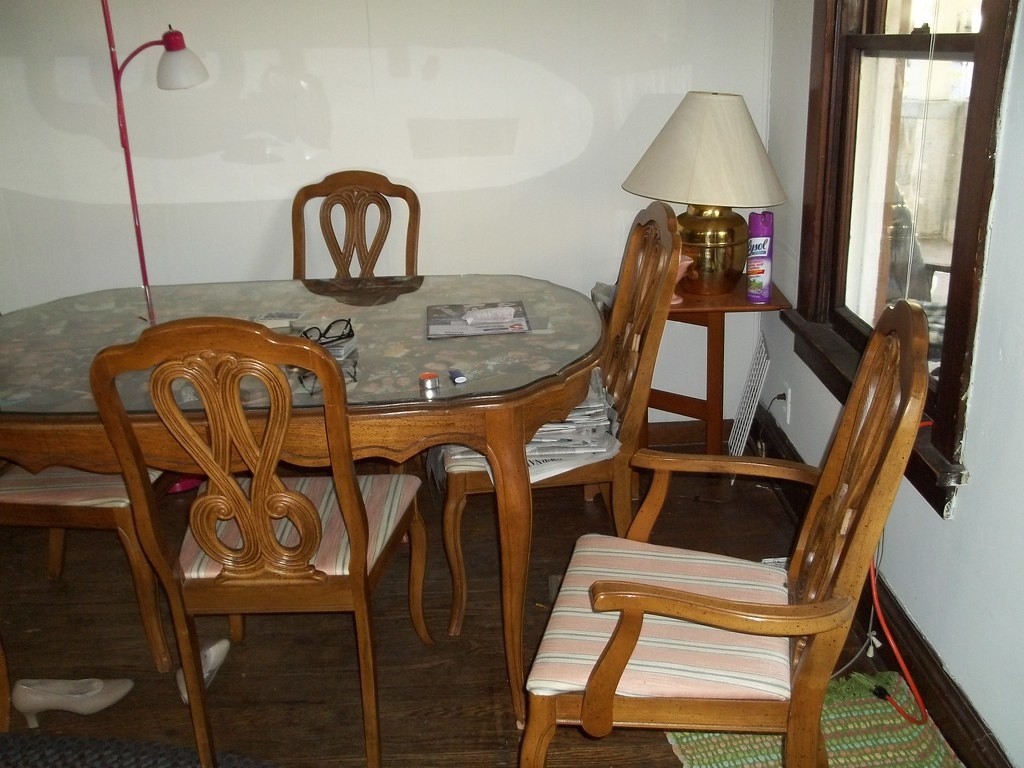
[621,90,785,296]
[100,0,208,286]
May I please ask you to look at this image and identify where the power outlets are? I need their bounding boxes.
[781,382,791,424]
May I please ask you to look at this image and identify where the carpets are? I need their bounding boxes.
[662,669,968,768]
[0,730,278,768]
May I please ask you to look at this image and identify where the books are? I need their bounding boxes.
[427,300,532,339]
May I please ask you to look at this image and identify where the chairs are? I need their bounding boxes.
[515,297,931,768]
[278,171,429,551]
[443,202,682,635]
[89,316,434,768]
[0,461,178,733]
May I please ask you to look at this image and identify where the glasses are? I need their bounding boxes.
[299,317,354,346]
[299,360,358,395]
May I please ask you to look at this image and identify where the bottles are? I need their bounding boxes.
[747,211,774,305]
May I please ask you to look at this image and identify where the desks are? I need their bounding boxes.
[621,271,793,457]
[0,274,605,732]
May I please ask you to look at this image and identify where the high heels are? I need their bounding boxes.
[11,678,134,729]
[177,639,231,704]
